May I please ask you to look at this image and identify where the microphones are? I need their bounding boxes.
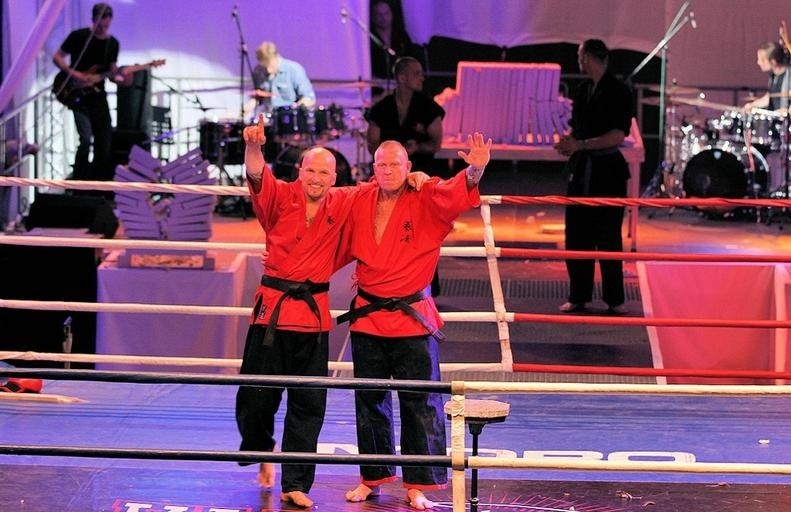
[341,7,347,25]
[231,2,238,20]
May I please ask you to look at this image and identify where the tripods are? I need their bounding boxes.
[628,2,791,230]
[208,149,236,202]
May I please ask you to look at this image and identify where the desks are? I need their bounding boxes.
[434,115,645,251]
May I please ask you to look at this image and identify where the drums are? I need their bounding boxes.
[314,108,343,138]
[200,118,245,164]
[272,105,308,142]
[718,110,783,143]
[682,146,769,214]
[270,144,352,187]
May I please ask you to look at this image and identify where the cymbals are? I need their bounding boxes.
[640,84,725,110]
[339,81,384,86]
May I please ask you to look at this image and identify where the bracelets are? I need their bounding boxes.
[582,139,586,149]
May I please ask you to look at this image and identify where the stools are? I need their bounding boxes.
[444,399,510,510]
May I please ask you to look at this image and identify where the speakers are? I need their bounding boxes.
[1,227,104,369]
[111,127,151,165]
[26,193,119,239]
[117,65,151,130]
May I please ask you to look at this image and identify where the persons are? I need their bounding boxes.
[244,41,316,115]
[234,111,430,507]
[555,40,636,311]
[363,56,451,298]
[744,40,791,115]
[53,3,130,180]
[260,133,491,509]
[780,20,791,52]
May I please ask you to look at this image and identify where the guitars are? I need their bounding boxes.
[52,60,164,106]
